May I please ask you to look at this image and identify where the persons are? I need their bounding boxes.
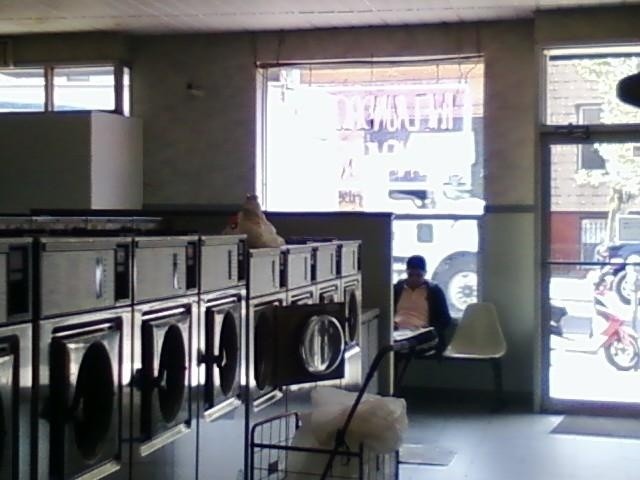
[395,256,451,357]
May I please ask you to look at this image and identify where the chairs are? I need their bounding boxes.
[403,302,508,413]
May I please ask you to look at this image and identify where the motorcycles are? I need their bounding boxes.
[549,265,640,370]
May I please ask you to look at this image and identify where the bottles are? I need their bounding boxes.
[240,193,261,247]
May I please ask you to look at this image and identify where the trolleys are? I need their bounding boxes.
[245,341,436,480]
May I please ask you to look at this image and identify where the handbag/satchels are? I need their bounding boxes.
[221,195,285,248]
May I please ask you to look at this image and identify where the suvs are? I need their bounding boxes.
[594,209,639,304]
[341,158,485,317]
